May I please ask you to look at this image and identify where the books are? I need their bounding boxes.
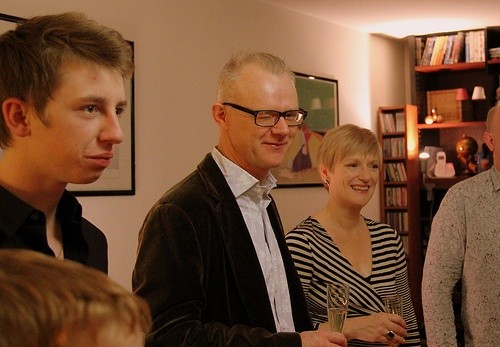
[386,187,407,207]
[415,31,485,67]
[402,236,408,256]
[387,212,408,231]
[384,137,406,157]
[384,162,407,181]
[380,113,406,132]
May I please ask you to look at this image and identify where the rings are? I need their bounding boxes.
[387,331,394,337]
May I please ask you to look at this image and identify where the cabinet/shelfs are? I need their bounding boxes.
[378,104,422,310]
[407,25,500,190]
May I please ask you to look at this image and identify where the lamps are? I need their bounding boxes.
[471,85,487,120]
[418,151,430,187]
[456,88,469,122]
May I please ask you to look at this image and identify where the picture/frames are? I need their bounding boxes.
[426,89,463,123]
[0,13,136,197]
[272,71,339,189]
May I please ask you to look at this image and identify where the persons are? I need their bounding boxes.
[421,102,500,347]
[0,11,131,277]
[285,124,420,347]
[132,52,349,347]
[0,248,151,347]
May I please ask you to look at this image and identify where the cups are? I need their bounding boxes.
[385,295,403,347]
[327,284,350,333]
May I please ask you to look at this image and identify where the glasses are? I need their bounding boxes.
[222,103,308,127]
